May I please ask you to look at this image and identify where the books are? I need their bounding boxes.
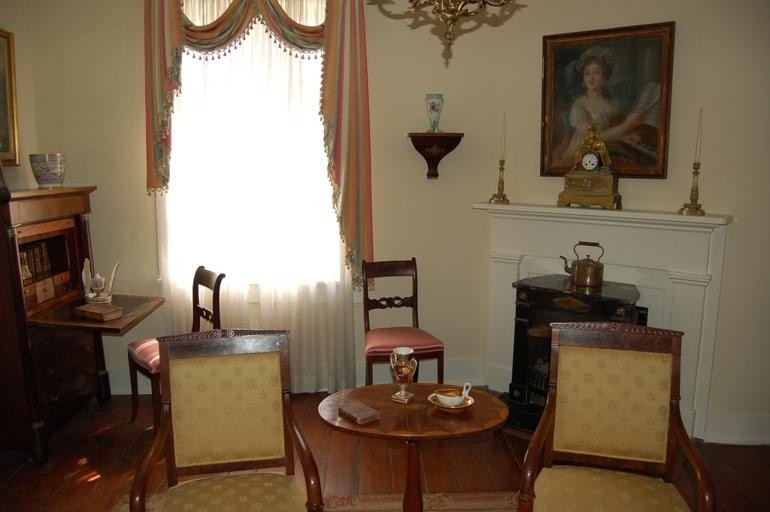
[20,251,32,279]
[338,399,381,425]
[27,248,36,276]
[39,242,51,274]
[32,247,43,274]
[626,82,662,130]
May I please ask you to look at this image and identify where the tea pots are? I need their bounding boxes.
[560,240,604,287]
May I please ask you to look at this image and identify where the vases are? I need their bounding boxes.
[421,92,451,135]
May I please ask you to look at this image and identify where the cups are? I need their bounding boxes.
[434,382,471,405]
[28,153,66,189]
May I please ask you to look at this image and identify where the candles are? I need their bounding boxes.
[496,116,512,160]
[693,107,708,162]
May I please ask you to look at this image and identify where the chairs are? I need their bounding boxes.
[124,326,329,512]
[509,321,719,510]
[121,262,227,427]
[360,256,447,389]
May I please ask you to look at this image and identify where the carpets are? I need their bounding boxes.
[300,488,538,512]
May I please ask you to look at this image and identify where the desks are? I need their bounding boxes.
[26,286,168,335]
[314,379,516,512]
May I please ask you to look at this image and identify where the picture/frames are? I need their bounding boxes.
[538,20,677,179]
[0,26,18,166]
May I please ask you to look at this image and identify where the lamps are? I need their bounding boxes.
[408,1,514,51]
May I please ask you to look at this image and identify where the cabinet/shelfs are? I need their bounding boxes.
[507,271,652,439]
[2,185,113,462]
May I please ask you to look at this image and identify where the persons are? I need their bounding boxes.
[550,44,646,171]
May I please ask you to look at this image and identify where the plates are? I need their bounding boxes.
[428,392,475,414]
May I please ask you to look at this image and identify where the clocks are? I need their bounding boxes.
[555,125,624,211]
